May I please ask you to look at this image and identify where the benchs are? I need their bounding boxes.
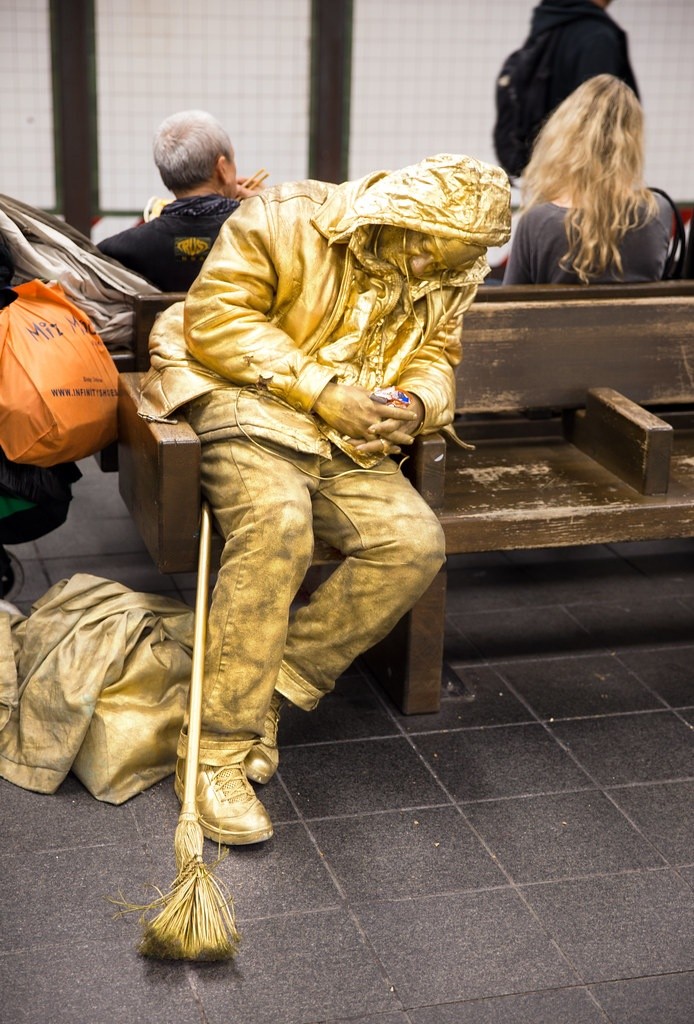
[105,275,694,716]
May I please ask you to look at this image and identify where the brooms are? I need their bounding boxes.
[108,499,243,964]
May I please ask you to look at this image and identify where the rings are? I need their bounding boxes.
[380,438,391,457]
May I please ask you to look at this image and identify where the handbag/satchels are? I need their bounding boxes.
[0,445,83,544]
[0,278,119,469]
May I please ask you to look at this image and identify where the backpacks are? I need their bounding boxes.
[493,20,564,175]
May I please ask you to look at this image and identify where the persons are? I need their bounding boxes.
[492,0,641,173]
[173,152,510,843]
[94,109,262,366]
[503,73,671,285]
